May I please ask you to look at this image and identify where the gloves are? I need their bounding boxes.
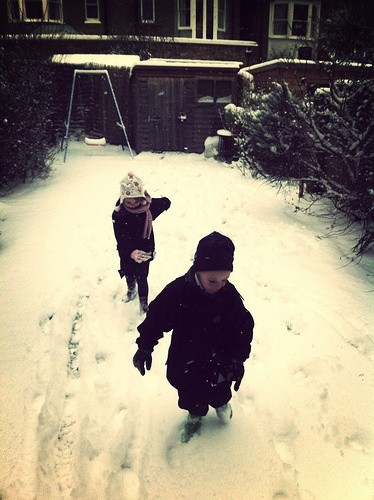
[133,347,154,376]
[226,358,245,392]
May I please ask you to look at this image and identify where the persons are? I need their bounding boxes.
[132,232,256,442]
[111,173,171,314]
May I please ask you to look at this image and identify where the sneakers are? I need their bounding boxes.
[122,289,149,318]
[180,401,232,444]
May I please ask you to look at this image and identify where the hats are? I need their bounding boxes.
[193,231,235,272]
[113,172,152,213]
